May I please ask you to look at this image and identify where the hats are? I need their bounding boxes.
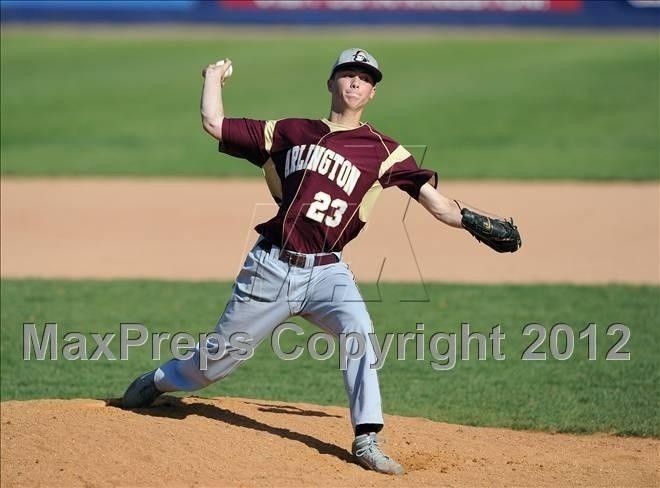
[330,47,383,85]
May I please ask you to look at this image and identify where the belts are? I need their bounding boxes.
[255,239,339,270]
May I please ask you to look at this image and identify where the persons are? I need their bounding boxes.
[122,47,522,475]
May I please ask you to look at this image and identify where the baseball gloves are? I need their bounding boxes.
[460,208,522,253]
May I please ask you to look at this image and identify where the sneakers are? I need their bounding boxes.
[121,370,165,410]
[351,432,404,476]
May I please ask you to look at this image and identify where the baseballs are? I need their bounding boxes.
[216,60,232,78]
